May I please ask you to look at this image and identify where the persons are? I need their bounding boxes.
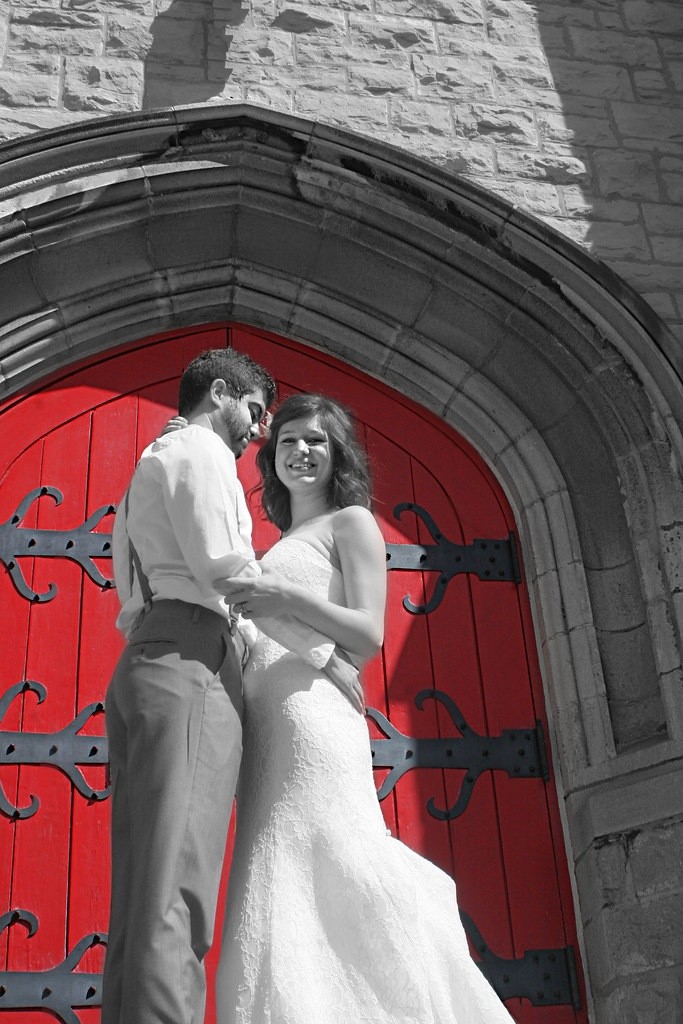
[98,347,365,1024]
[162,395,519,1024]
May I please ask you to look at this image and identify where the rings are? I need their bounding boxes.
[242,602,248,615]
